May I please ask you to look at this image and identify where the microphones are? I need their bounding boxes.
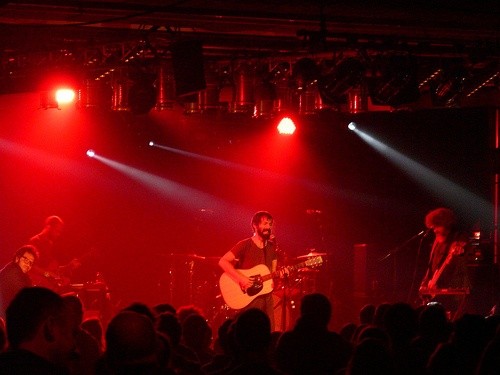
[270,235,276,243]
[424,221,440,239]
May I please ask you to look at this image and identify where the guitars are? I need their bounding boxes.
[428,241,465,299]
[219,254,323,310]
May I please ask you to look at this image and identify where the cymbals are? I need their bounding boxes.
[298,253,327,259]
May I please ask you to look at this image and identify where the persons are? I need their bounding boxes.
[30,215,81,281]
[219,211,289,333]
[0,245,40,318]
[0,285,500,375]
[418,207,472,320]
